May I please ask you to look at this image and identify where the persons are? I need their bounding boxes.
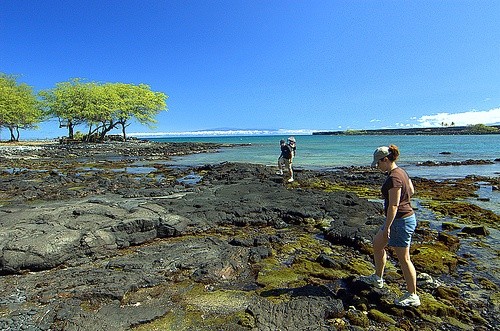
[275,136,297,182]
[360,145,421,307]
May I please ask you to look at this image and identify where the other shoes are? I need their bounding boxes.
[275,172,283,175]
[287,179,294,182]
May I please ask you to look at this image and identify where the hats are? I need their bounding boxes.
[288,136,296,143]
[279,139,285,145]
[371,146,392,167]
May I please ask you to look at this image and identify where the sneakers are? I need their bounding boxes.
[360,274,383,289]
[394,292,420,307]
